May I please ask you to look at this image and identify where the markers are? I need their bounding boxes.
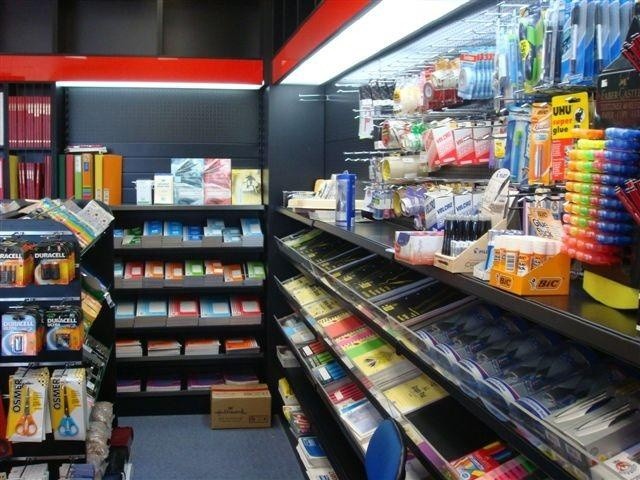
[555,26,561,81]
[569,5,578,74]
[542,27,553,84]
[596,6,604,72]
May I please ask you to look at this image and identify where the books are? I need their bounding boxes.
[114,260,267,288]
[225,338,260,355]
[230,297,261,325]
[199,298,230,325]
[225,374,259,385]
[136,297,167,327]
[114,301,135,328]
[146,378,181,392]
[282,275,449,416]
[284,227,478,327]
[168,299,198,326]
[184,338,220,355]
[0,154,51,199]
[147,340,181,357]
[187,377,224,391]
[136,157,262,205]
[114,219,264,247]
[295,437,340,480]
[66,144,107,152]
[116,339,143,358]
[7,96,51,148]
[58,152,122,206]
[117,379,140,392]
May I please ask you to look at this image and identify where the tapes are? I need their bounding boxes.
[430,73,440,87]
[458,67,471,88]
[422,81,435,102]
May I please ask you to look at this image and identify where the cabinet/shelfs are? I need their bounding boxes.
[0,199,117,476]
[272,0,639,479]
[111,201,270,415]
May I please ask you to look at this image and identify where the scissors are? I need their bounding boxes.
[14,386,38,437]
[58,386,78,437]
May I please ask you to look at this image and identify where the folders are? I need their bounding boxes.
[94,154,103,203]
[66,154,74,199]
[57,154,65,198]
[82,153,94,200]
[103,154,123,206]
[74,155,83,199]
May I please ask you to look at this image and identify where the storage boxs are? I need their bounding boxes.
[209,386,270,428]
